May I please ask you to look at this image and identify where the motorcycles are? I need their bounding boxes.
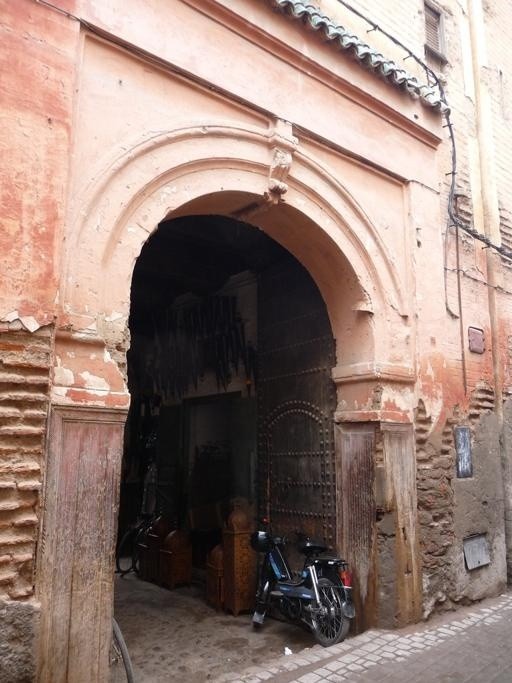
[249,516,356,647]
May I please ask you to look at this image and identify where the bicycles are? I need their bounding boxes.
[112,617,135,682]
[116,507,164,573]
